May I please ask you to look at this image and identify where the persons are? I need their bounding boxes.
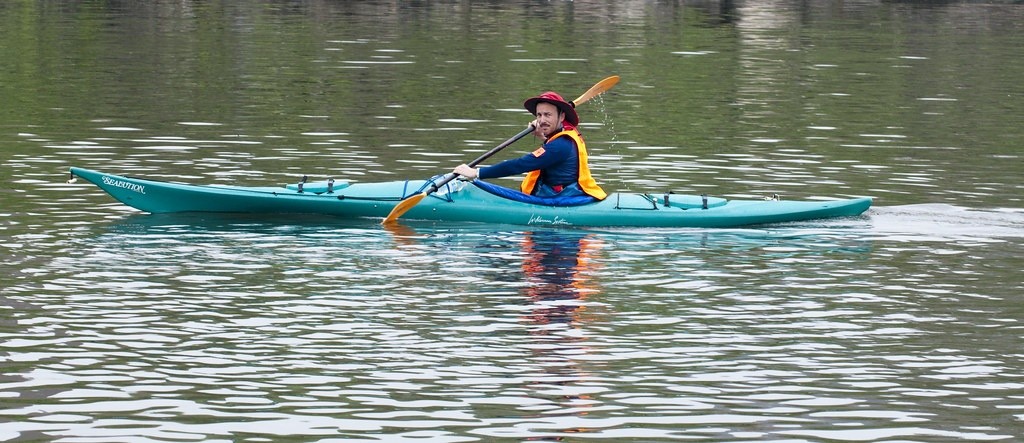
[453,92,607,201]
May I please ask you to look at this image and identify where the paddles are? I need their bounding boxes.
[380,74,620,224]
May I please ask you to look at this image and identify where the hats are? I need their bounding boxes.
[524,91,578,127]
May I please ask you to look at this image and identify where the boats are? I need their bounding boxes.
[101,216,868,251]
[63,165,874,217]
